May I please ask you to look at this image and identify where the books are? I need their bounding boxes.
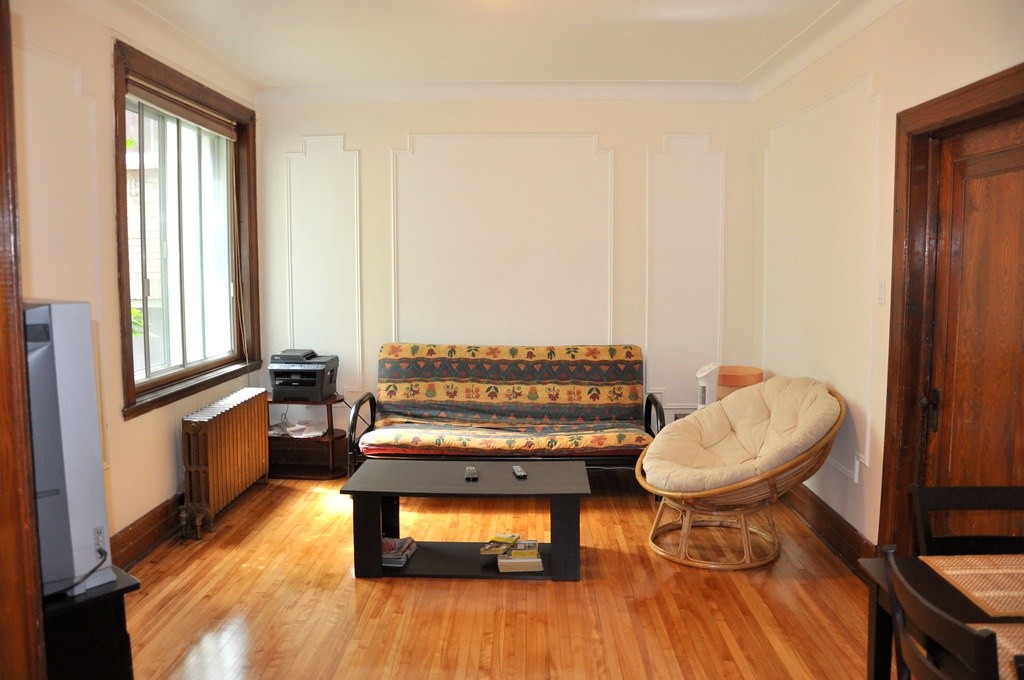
[382,537,417,568]
[480,534,544,573]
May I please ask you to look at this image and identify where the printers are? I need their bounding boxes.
[268,348,339,402]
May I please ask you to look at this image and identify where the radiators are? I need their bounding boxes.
[178,386,269,539]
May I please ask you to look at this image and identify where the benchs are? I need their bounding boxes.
[348,343,665,502]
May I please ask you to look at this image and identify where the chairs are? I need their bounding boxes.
[880,543,1000,680]
[635,375,847,570]
[911,483,1024,556]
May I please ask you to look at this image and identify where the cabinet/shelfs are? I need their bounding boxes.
[267,392,348,479]
[43,563,143,680]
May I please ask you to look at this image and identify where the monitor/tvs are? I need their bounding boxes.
[22,301,117,597]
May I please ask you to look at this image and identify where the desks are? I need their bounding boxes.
[855,554,1024,680]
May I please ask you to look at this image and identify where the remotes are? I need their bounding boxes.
[512,466,528,478]
[464,466,478,481]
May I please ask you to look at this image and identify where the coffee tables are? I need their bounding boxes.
[340,458,591,581]
